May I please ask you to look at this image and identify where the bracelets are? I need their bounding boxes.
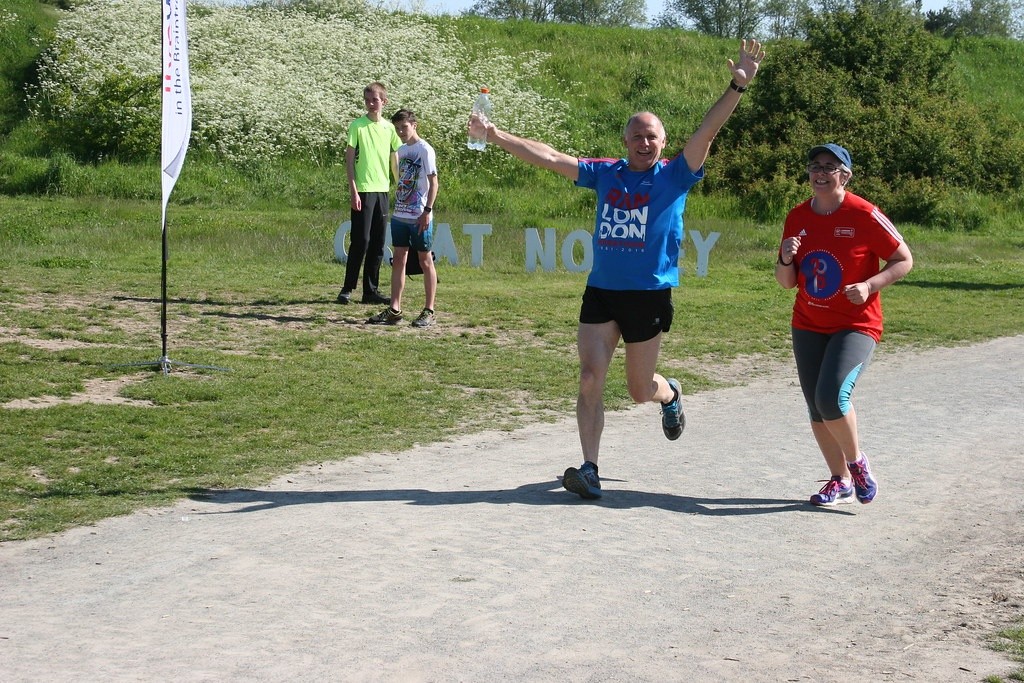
[730,80,746,93]
[424,207,431,212]
[779,255,793,266]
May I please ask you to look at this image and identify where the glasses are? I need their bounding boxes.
[807,163,846,174]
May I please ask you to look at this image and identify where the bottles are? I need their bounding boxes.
[467,88,490,151]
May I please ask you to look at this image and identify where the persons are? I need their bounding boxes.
[337,82,404,306]
[467,38,766,500]
[369,109,438,326]
[774,144,913,506]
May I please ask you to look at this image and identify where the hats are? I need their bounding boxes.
[809,143,851,169]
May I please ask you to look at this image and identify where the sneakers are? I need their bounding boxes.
[659,377,686,441]
[369,309,403,325]
[412,310,436,329]
[846,451,877,504]
[562,464,602,499]
[810,476,853,506]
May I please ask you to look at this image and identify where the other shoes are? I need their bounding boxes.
[362,290,390,304]
[337,288,352,303]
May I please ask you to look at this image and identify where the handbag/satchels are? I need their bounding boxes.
[390,245,435,275]
